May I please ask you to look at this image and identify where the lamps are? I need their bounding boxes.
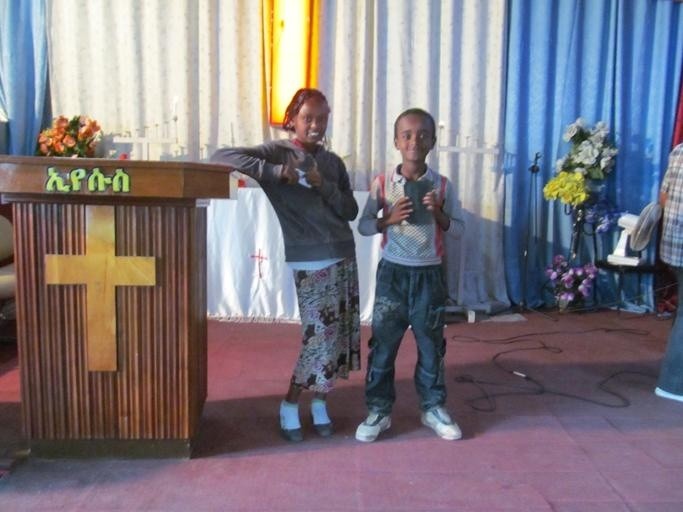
[258,0,324,129]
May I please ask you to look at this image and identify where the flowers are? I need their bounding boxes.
[555,117,619,179]
[542,170,589,206]
[38,114,101,157]
[583,200,630,234]
[543,254,599,311]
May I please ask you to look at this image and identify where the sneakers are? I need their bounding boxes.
[419,404,464,442]
[353,410,395,442]
[308,398,335,438]
[276,402,305,443]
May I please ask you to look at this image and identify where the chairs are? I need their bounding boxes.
[592,221,662,318]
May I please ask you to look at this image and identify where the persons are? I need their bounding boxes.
[212,88,361,443]
[355,109,466,442]
[655,142,683,402]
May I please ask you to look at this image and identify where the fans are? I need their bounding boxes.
[607,201,662,267]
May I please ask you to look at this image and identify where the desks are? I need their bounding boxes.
[0,156,236,462]
